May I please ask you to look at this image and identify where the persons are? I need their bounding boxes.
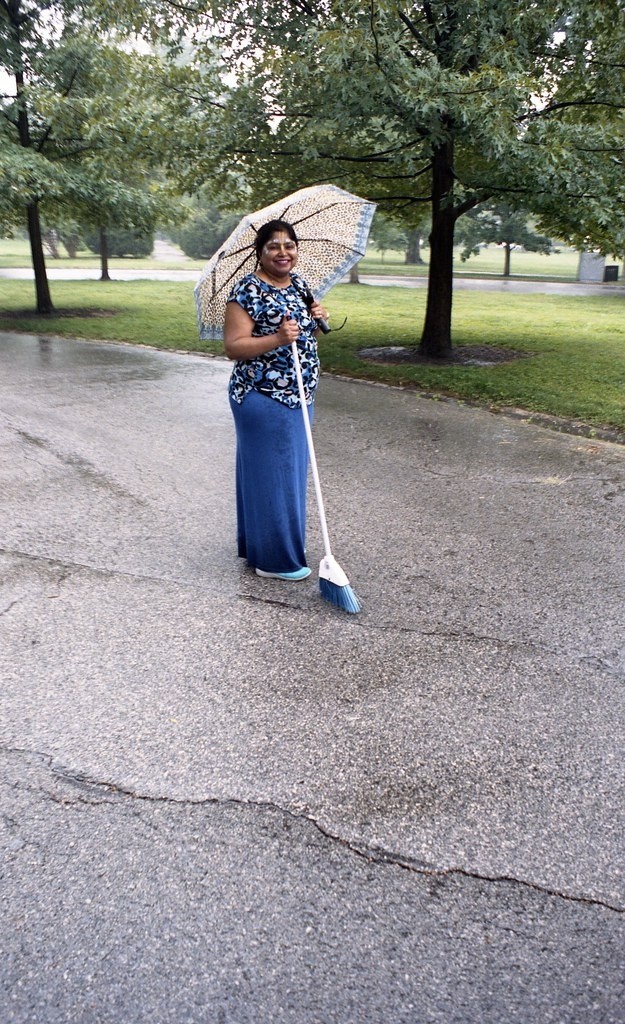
[224,220,330,581]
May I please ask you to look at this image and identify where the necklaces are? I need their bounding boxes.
[269,276,292,286]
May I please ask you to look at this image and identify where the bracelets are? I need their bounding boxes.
[327,313,330,319]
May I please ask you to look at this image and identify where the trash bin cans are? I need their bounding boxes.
[605,265,619,282]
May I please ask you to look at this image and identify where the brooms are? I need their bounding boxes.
[284,316,362,614]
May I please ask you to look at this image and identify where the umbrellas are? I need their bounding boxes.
[193,184,380,340]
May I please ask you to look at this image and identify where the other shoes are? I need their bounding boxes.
[255,567,311,581]
